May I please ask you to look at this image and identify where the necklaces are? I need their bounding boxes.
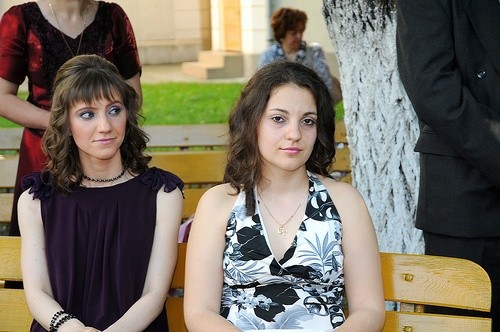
[83,167,127,183]
[257,182,309,240]
[48,0,90,57]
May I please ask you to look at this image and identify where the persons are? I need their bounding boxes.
[16,53,183,332]
[183,60,385,332]
[255,7,335,127]
[1,0,144,289]
[395,1,500,331]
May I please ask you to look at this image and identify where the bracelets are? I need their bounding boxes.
[47,310,79,332]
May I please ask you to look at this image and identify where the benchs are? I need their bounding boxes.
[0,120,352,225]
[0,234,492,332]
